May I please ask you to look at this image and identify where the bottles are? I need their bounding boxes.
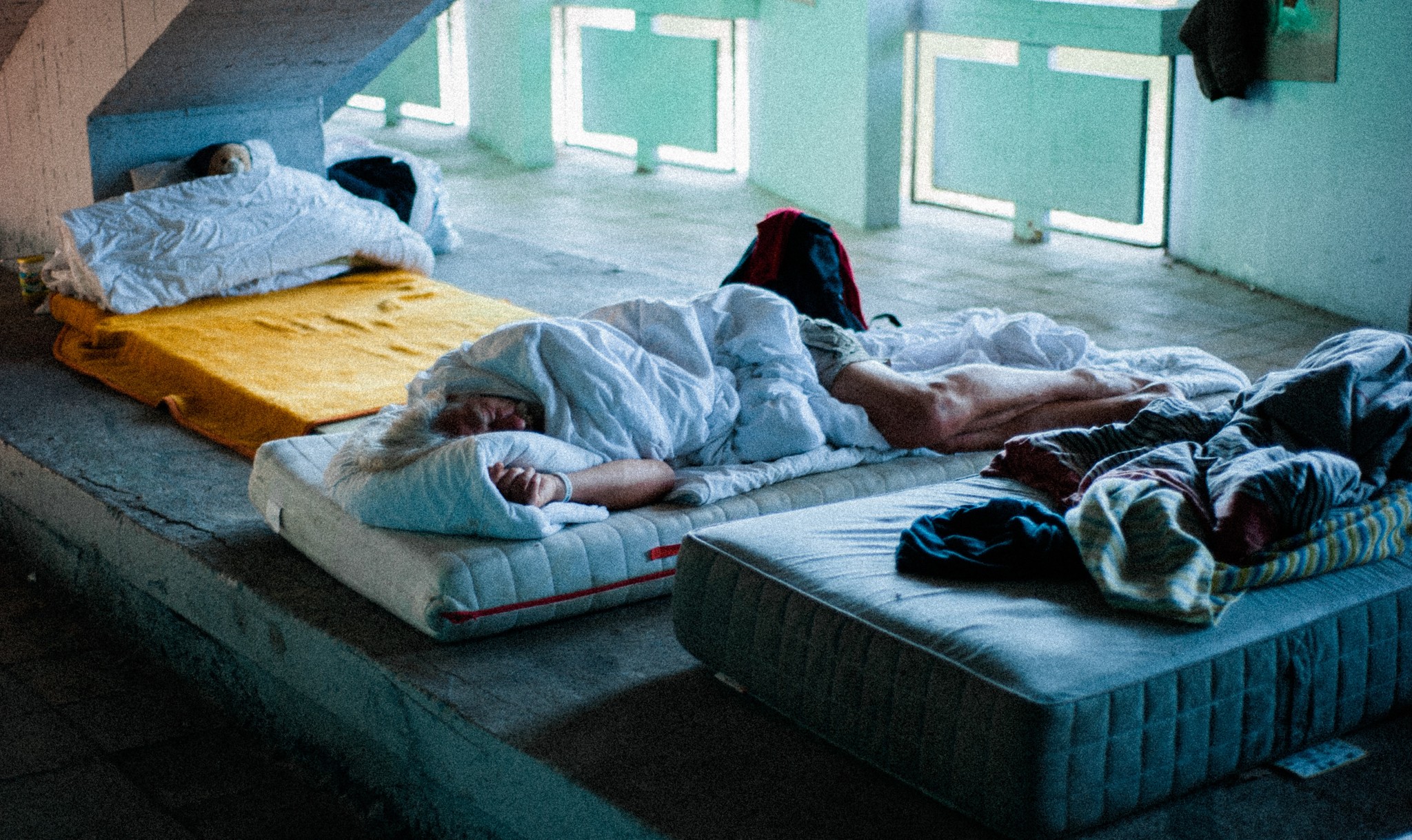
[17,254,51,304]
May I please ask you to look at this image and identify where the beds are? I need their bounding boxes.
[249,322,1246,642]
[670,475,1412,840]
[48,267,551,463]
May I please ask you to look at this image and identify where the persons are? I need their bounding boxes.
[377,313,1187,508]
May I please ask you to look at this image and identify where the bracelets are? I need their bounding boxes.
[550,470,573,505]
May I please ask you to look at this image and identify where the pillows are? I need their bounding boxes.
[129,138,277,192]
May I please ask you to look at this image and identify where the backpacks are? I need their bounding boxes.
[719,206,869,333]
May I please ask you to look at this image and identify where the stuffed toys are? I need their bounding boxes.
[210,144,251,180]
